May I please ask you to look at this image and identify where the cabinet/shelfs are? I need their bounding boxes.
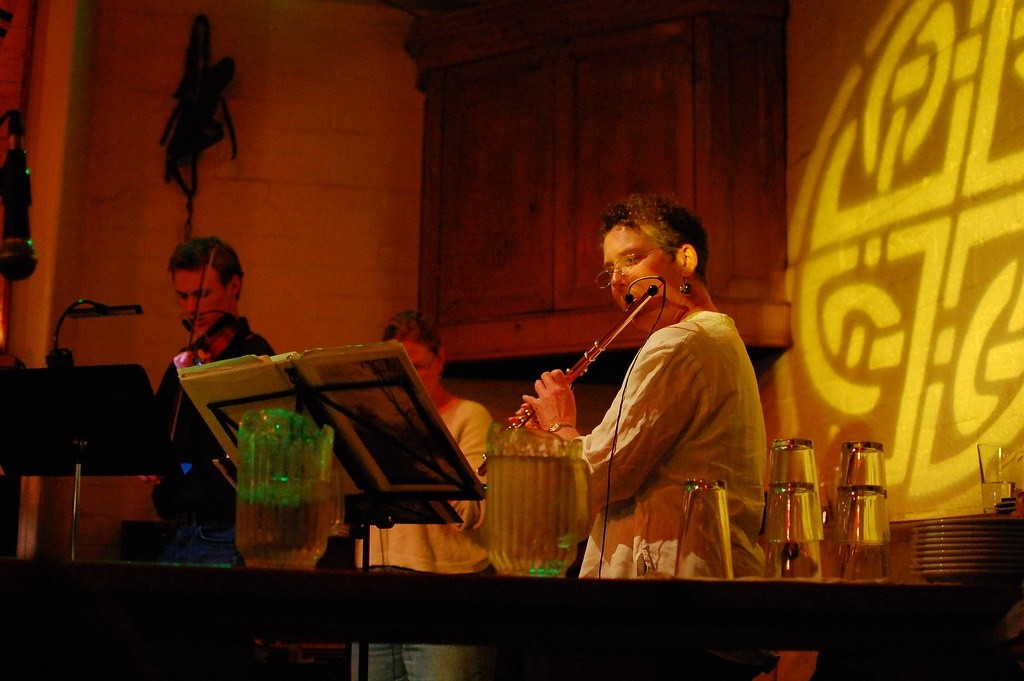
[403,0,795,384]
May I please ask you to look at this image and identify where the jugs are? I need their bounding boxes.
[235,409,334,571]
[484,423,587,578]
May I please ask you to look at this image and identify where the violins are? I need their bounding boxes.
[185,313,239,365]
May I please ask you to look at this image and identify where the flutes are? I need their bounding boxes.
[475,285,658,477]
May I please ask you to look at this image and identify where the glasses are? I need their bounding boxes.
[414,354,435,375]
[594,245,679,289]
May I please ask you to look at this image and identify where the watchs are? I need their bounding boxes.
[548,423,575,433]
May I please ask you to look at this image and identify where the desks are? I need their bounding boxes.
[0,559,1024,681]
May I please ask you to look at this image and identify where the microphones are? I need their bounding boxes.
[625,276,665,304]
[0,112,38,281]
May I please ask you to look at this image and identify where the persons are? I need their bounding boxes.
[136,235,275,564]
[351,308,493,681]
[508,190,768,581]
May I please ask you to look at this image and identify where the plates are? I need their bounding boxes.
[909,518,1024,583]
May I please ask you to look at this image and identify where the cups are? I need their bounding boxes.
[837,484,891,583]
[764,439,824,543]
[768,481,822,580]
[834,441,890,545]
[978,443,1015,514]
[675,479,734,580]
